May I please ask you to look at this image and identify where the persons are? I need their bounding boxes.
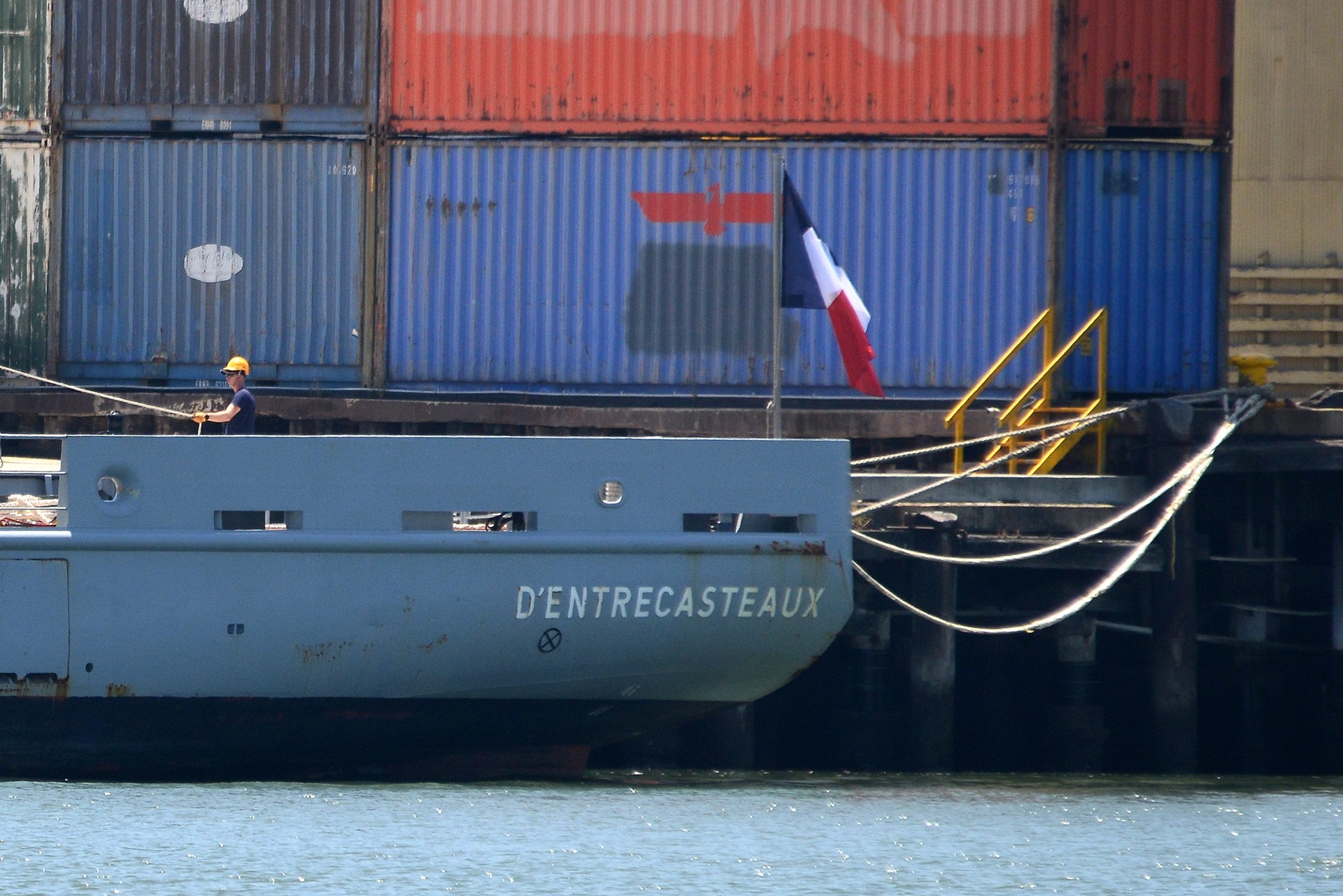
[191,356,256,436]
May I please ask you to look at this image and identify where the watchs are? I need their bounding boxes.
[204,412,210,422]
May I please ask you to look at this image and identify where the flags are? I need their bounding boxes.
[777,167,887,403]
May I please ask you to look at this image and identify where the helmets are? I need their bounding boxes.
[220,356,249,376]
